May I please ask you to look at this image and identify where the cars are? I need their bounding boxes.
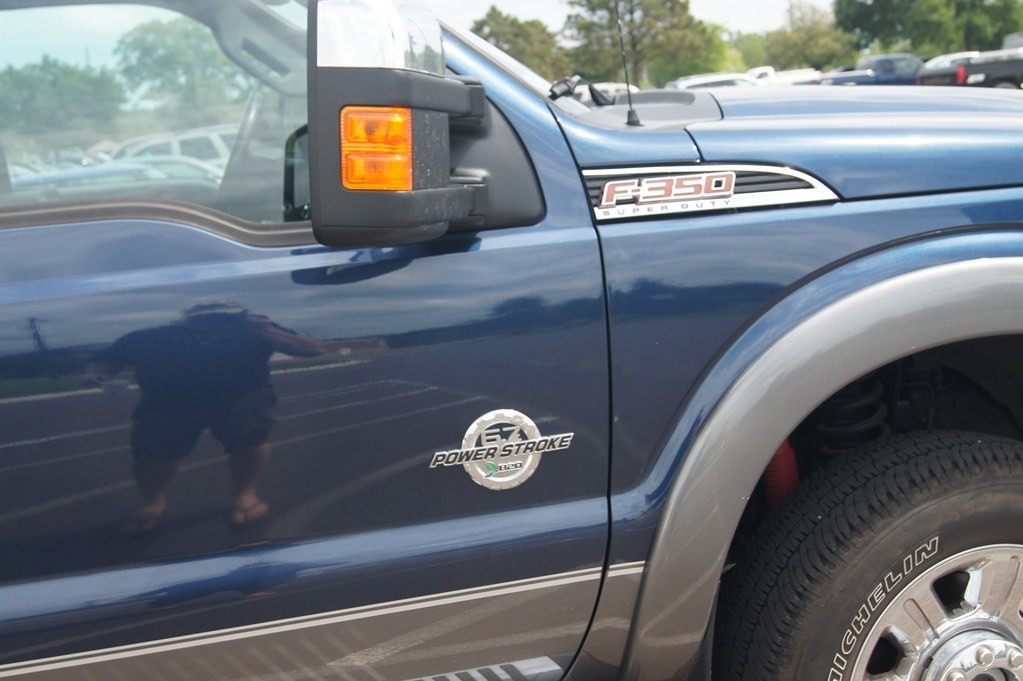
[550,40,1023,107]
[5,109,257,202]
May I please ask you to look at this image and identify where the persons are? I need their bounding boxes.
[90,300,325,543]
[447,272,688,505]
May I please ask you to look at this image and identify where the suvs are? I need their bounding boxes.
[2,0,1023,681]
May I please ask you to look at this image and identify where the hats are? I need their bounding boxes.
[184,299,246,319]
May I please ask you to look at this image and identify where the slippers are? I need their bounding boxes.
[118,510,166,535]
[232,501,268,529]
